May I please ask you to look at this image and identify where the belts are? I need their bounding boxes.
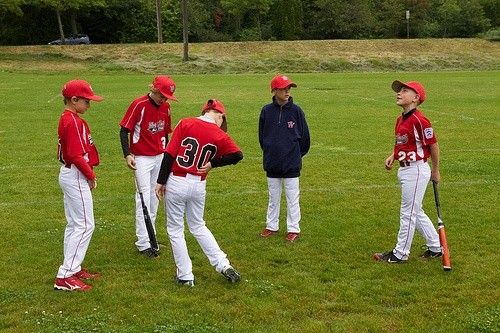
[172,171,205,180]
[65,163,71,168]
[399,158,427,167]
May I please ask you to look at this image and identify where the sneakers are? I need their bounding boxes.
[73,268,101,279]
[374,250,406,263]
[259,229,279,237]
[418,249,442,260]
[285,232,300,241]
[54,274,93,292]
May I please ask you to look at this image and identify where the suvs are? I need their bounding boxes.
[47,34,90,45]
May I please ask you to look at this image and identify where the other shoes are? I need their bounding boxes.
[156,241,166,250]
[139,248,160,258]
[175,275,195,286]
[221,265,241,282]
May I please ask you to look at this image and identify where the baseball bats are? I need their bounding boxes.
[132,171,159,252]
[432,179,452,272]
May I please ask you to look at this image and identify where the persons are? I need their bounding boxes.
[258,73,310,242]
[119,74,179,259]
[53,80,106,293]
[156,99,243,286]
[375,81,440,264]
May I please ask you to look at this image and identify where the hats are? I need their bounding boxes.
[153,75,177,101]
[270,73,297,91]
[62,79,103,101]
[391,79,426,105]
[201,98,227,133]
